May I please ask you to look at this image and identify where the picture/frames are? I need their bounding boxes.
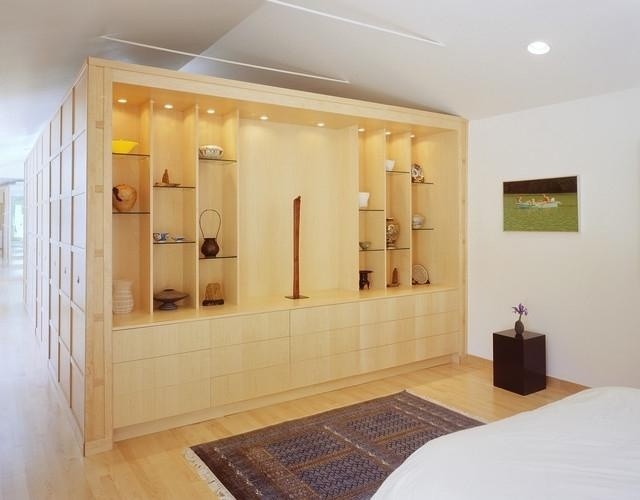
[500,174,581,234]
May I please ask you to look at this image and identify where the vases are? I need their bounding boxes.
[515,319,524,335]
[385,216,400,250]
[111,181,137,212]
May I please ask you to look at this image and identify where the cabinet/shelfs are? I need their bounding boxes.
[86,58,468,456]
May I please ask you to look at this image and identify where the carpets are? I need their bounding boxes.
[185,389,486,500]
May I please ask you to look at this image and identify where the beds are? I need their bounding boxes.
[374,386,640,498]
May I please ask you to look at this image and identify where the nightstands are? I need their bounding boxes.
[493,328,546,396]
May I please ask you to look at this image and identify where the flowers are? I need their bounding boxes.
[511,303,527,319]
